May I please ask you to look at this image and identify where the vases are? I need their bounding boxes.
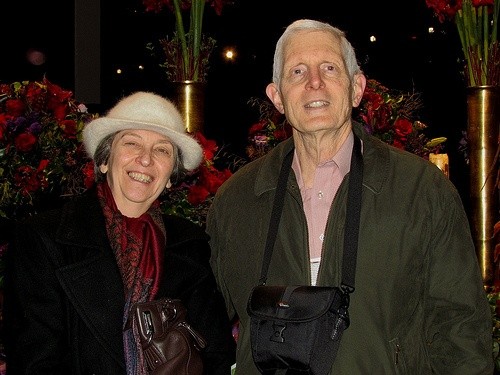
[465,85,500,283]
[172,79,203,135]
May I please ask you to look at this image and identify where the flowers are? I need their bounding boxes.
[0,0,500,375]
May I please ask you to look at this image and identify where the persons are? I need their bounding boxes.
[4,91,237,375]
[205,19,495,375]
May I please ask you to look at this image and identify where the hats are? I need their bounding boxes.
[82,91,203,170]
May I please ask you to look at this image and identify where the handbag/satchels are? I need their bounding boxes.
[247,283,349,375]
[136,296,210,375]
